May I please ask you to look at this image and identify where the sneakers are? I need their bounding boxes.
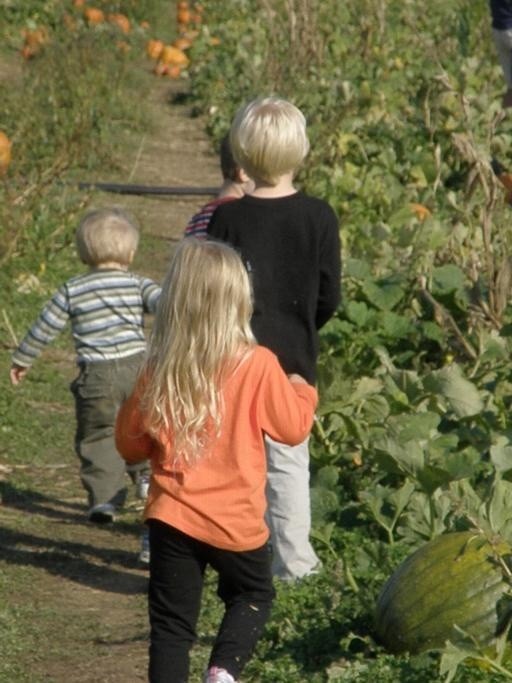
[89,502,115,520]
[201,668,240,683]
[137,551,151,563]
[135,476,150,497]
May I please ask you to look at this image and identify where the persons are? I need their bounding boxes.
[181,131,255,246]
[113,234,320,682]
[208,97,343,581]
[8,203,166,527]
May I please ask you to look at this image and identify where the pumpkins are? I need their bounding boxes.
[18,1,218,80]
[1,134,14,173]
[374,530,509,655]
[407,202,432,223]
